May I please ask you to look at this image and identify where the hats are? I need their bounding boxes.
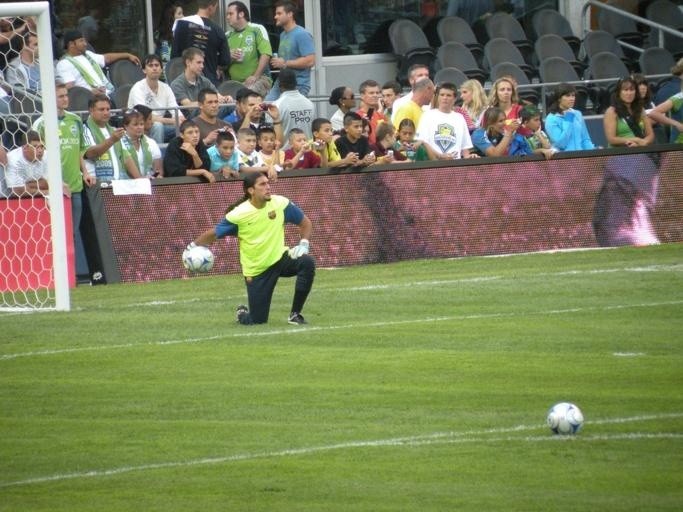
[62,30,84,50]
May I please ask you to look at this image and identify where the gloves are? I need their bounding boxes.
[180,241,199,269]
[287,238,310,261]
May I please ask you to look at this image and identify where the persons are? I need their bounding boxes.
[182,170,316,327]
[313,58,681,169]
[1,2,328,234]
[591,150,668,247]
[366,2,514,17]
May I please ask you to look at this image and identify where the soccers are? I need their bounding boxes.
[547,403,584,434]
[182,246,214,273]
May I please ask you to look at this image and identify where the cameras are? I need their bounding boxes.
[407,146,413,151]
[259,103,268,112]
[218,128,227,133]
[516,119,523,127]
[312,141,322,149]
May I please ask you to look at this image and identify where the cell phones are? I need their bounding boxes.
[388,151,393,159]
[116,121,122,128]
[381,99,386,107]
[272,53,277,58]
[370,151,375,159]
[153,172,159,177]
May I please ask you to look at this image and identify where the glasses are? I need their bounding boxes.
[124,108,137,115]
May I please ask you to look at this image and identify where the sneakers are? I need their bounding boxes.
[234,304,249,322]
[285,311,307,326]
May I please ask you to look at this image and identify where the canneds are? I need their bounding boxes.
[237,49,243,62]
[271,52,279,58]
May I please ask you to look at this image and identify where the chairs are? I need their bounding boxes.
[388,0,682,112]
[0,29,263,139]
